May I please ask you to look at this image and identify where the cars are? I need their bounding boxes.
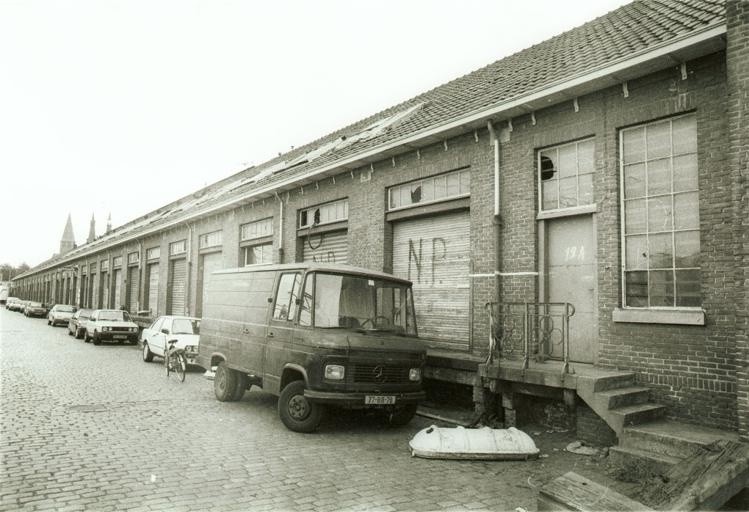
[141,314,201,372]
[5,297,139,346]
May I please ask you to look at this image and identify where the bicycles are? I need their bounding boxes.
[151,332,187,382]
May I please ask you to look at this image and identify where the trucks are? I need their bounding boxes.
[198,261,427,432]
